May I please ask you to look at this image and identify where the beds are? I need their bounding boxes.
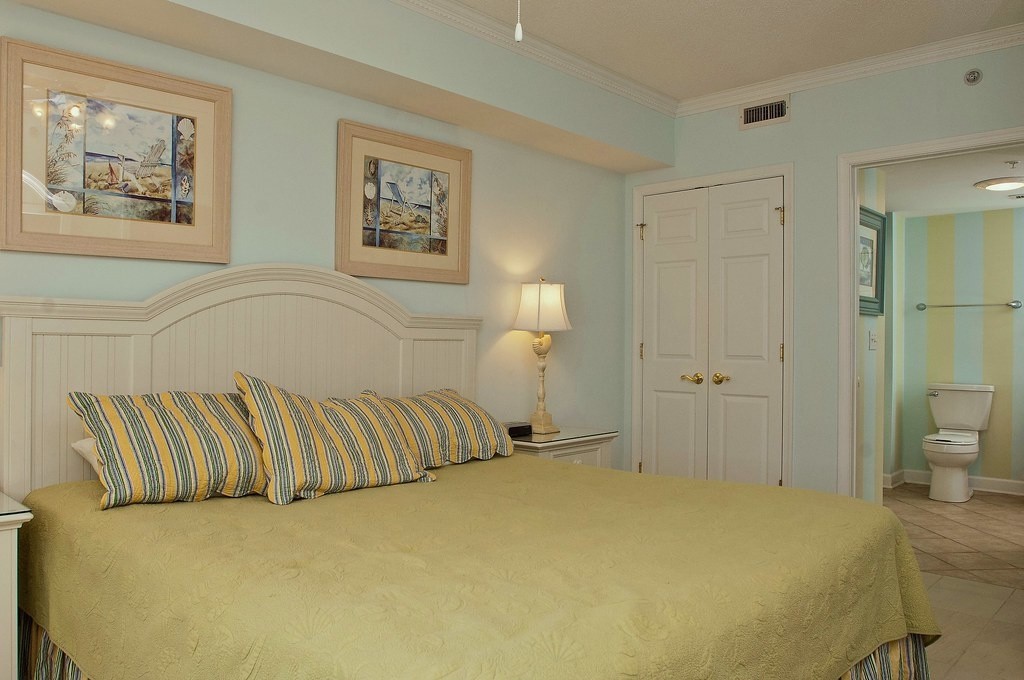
[0,262,942,680]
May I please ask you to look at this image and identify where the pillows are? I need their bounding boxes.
[67,385,266,510]
[232,366,438,508]
[383,384,515,469]
[69,437,102,487]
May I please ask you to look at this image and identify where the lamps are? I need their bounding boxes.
[973,176,1024,191]
[512,276,574,434]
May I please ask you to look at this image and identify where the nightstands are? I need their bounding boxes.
[509,433,619,466]
[0,492,35,680]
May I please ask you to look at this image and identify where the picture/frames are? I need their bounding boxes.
[855,202,887,314]
[0,35,234,266]
[335,118,472,287]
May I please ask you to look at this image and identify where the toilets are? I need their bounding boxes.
[920,382,996,503]
